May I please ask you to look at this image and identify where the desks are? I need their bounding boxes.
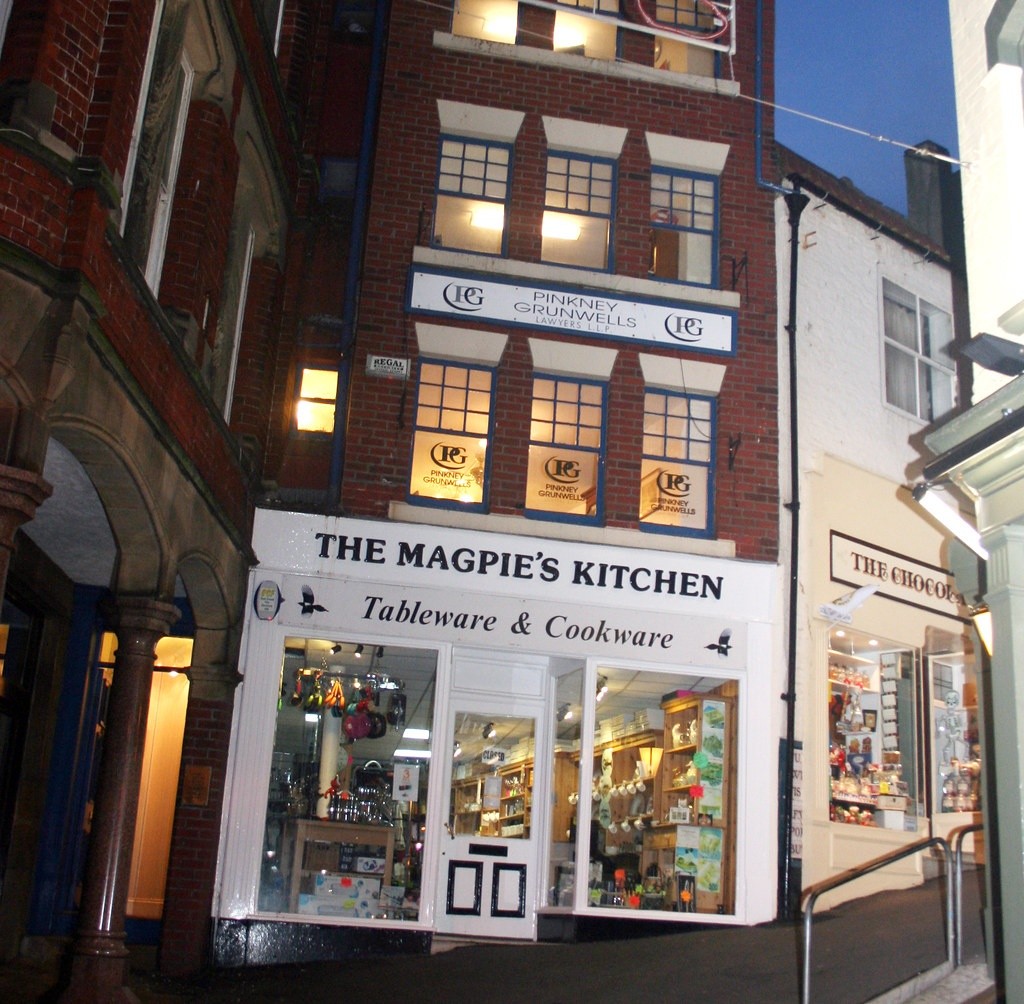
[280,816,398,913]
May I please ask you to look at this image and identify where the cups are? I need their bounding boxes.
[565,781,646,838]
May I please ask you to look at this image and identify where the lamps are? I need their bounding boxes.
[330,641,342,655]
[596,676,607,701]
[355,644,363,658]
[376,647,383,658]
[483,723,496,739]
[453,741,462,757]
[556,704,574,721]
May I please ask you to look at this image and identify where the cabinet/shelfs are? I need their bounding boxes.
[827,648,882,820]
[572,729,665,850]
[448,757,534,839]
[642,693,732,876]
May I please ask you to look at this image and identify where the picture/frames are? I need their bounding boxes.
[861,709,878,733]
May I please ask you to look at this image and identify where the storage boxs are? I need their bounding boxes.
[554,737,581,752]
[299,842,406,917]
[452,736,534,780]
[501,824,523,837]
[669,807,691,823]
[593,708,665,745]
